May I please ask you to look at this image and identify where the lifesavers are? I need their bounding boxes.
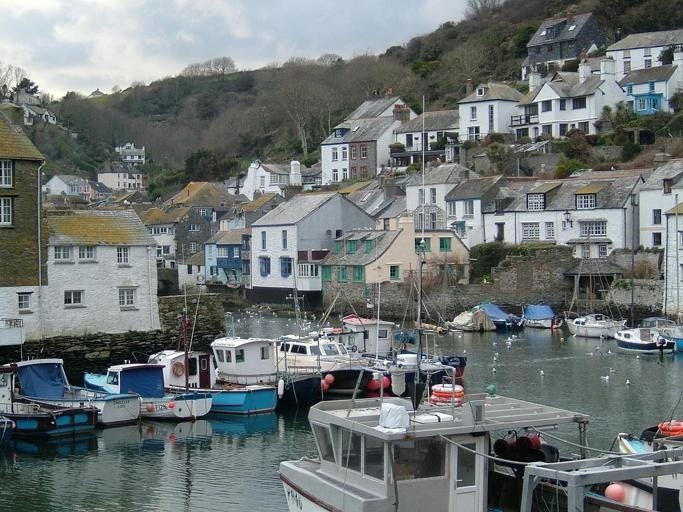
[659,419,683,436]
[172,362,185,376]
[431,384,464,408]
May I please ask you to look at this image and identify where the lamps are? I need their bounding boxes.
[562,210,572,227]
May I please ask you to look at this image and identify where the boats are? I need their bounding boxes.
[0,358,141,443]
[210,337,321,406]
[85,363,212,419]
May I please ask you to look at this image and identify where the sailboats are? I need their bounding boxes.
[145,244,279,413]
